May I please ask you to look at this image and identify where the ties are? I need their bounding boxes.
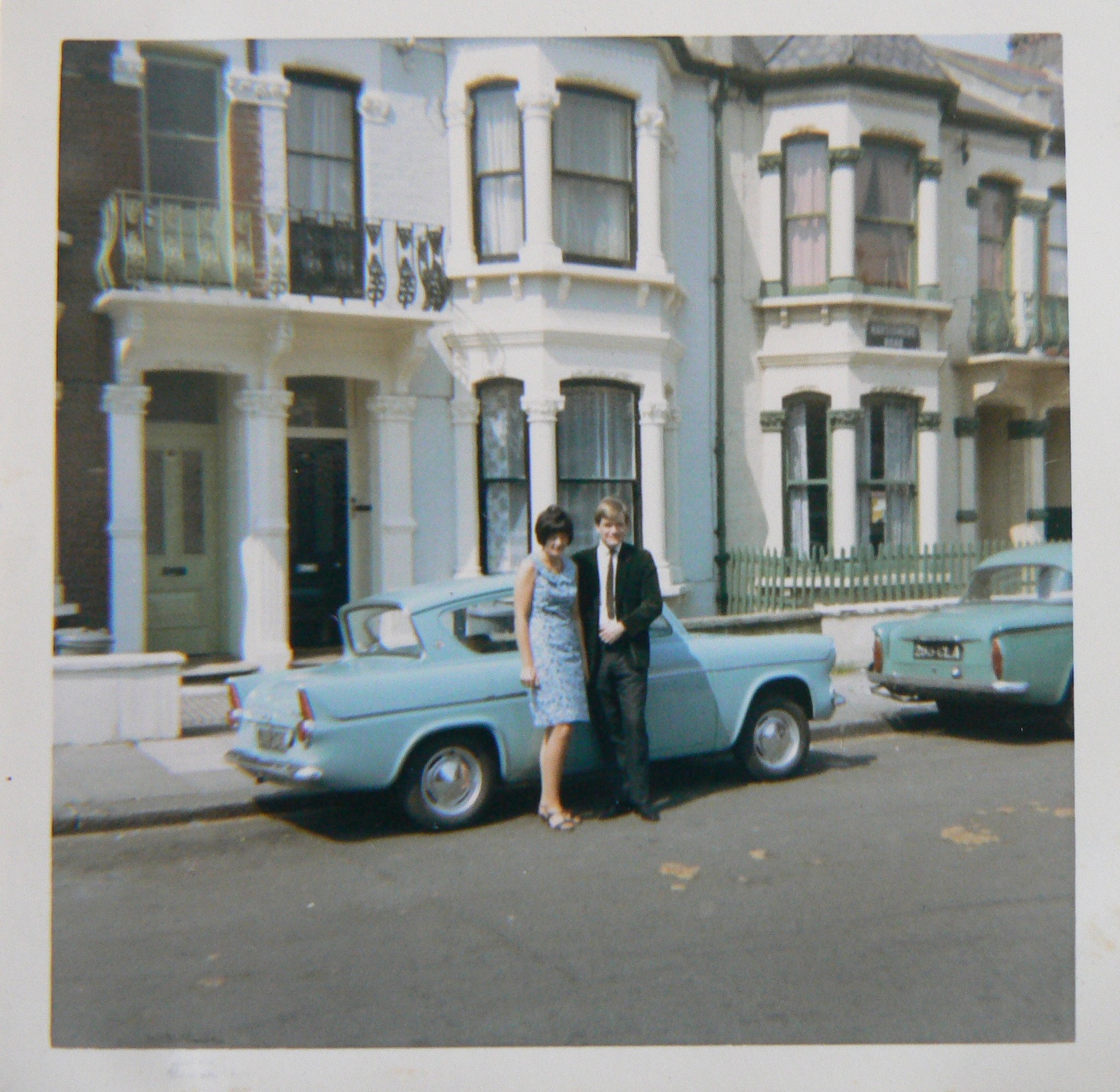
[606,550,617,619]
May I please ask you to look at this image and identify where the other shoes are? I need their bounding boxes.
[630,800,660,822]
[599,798,630,819]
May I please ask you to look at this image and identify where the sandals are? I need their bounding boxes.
[546,812,577,832]
[538,808,583,825]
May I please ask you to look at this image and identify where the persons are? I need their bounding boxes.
[570,496,662,821]
[513,504,590,831]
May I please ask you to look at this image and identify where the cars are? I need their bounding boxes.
[866,540,1075,740]
[221,571,847,834]
